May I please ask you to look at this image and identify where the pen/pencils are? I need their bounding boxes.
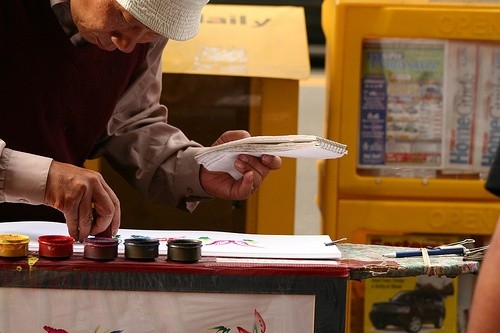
[382,247,464,257]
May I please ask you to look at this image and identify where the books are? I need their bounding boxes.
[196,132,347,181]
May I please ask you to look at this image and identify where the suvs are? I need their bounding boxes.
[369,288,446,333]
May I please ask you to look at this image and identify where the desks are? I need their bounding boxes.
[0,221,479,333]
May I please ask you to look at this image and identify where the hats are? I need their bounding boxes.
[117,0,211,41]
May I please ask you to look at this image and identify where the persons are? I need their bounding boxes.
[1,0,282,243]
[465,144,500,333]
